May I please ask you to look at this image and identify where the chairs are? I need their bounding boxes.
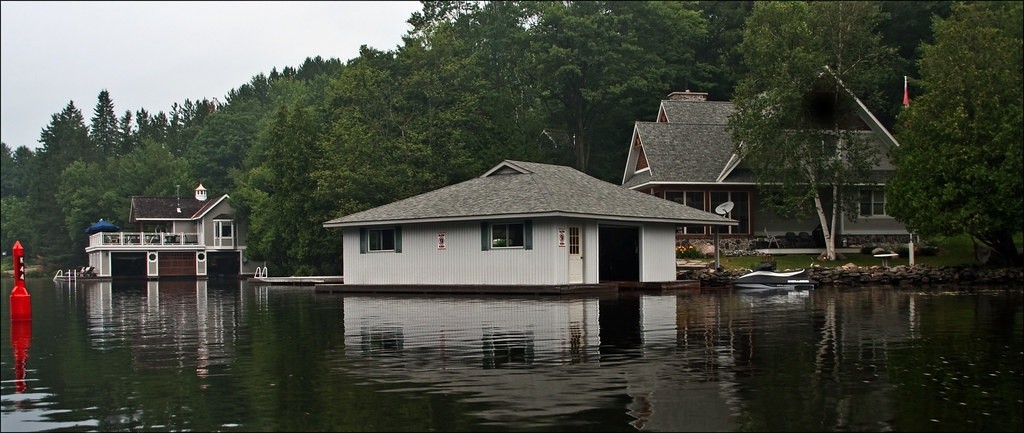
[65,266,97,279]
[108,236,118,242]
[763,227,780,249]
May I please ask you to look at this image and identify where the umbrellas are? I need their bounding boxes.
[85,218,121,233]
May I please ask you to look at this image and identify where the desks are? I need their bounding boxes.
[874,254,899,266]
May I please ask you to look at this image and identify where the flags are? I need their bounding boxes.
[903,80,909,105]
